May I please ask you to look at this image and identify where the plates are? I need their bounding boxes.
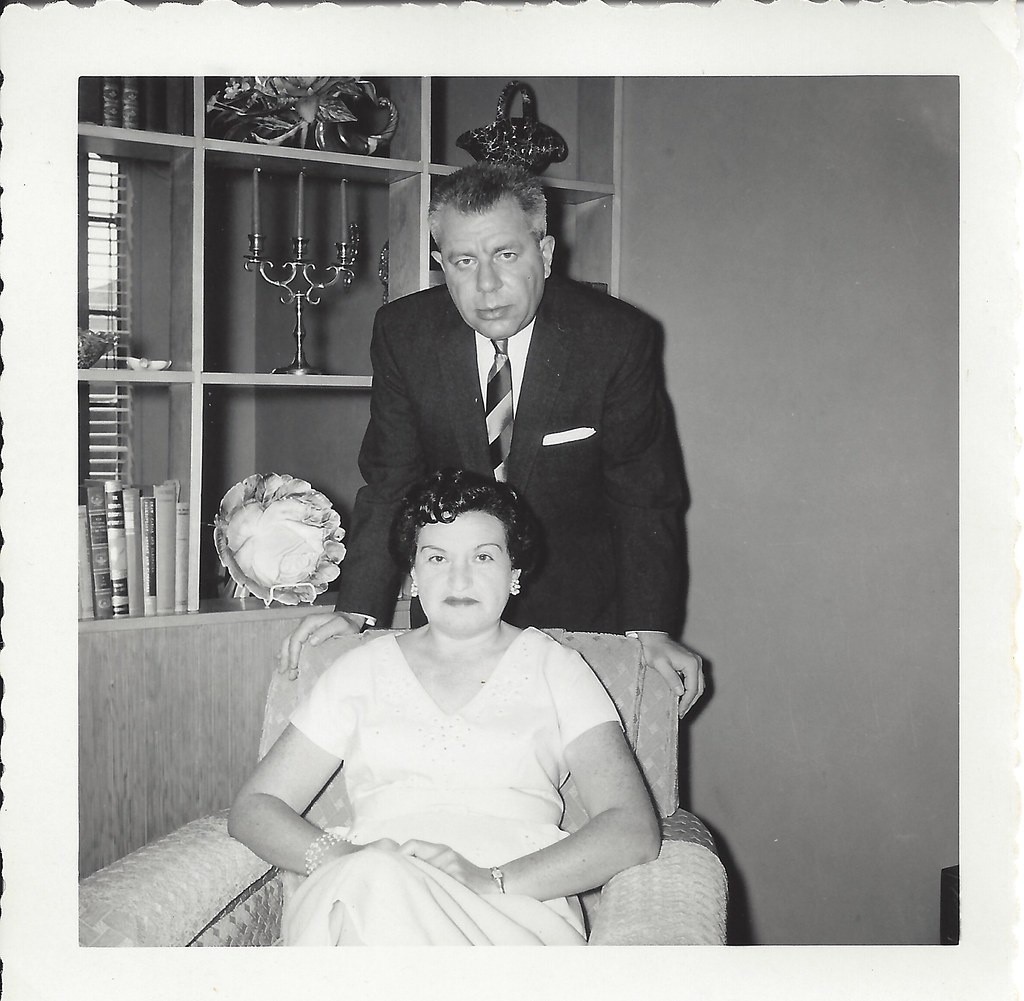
[213,473,345,607]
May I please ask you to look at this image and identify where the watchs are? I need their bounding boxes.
[490,866,506,893]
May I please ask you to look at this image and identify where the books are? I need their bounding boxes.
[78,76,191,132]
[78,476,191,617]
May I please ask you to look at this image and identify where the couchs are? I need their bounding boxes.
[78,622,727,947]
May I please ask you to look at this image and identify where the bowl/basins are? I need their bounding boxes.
[78,329,120,368]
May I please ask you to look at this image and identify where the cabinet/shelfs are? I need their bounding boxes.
[77,77,628,884]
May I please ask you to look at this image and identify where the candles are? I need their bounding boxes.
[251,166,260,236]
[297,171,304,240]
[341,179,348,243]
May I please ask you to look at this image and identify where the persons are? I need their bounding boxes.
[223,468,660,945]
[275,164,710,721]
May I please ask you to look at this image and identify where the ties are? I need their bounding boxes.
[483,338,514,483]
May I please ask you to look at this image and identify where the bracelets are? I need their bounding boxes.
[304,829,353,878]
